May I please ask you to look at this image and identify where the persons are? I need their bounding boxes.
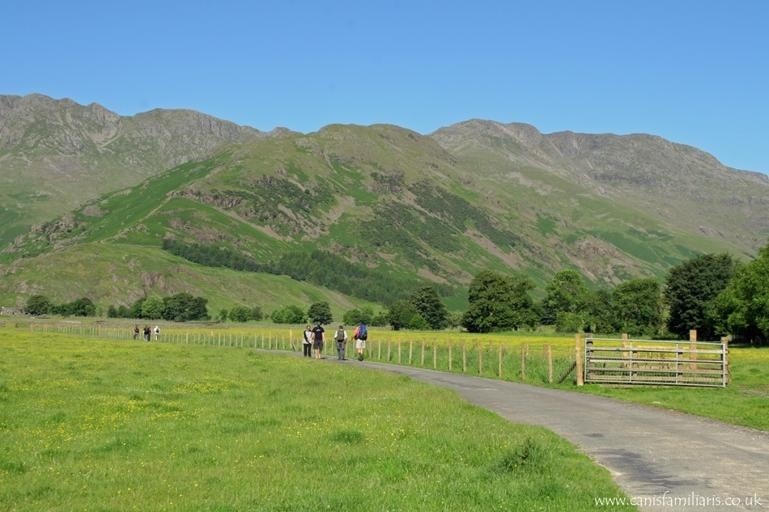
[350,322,367,361]
[334,325,348,360]
[310,322,326,358]
[142,323,150,341]
[131,324,140,339]
[154,325,161,340]
[302,325,313,356]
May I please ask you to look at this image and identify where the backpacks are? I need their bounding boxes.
[359,326,368,340]
[337,330,344,341]
